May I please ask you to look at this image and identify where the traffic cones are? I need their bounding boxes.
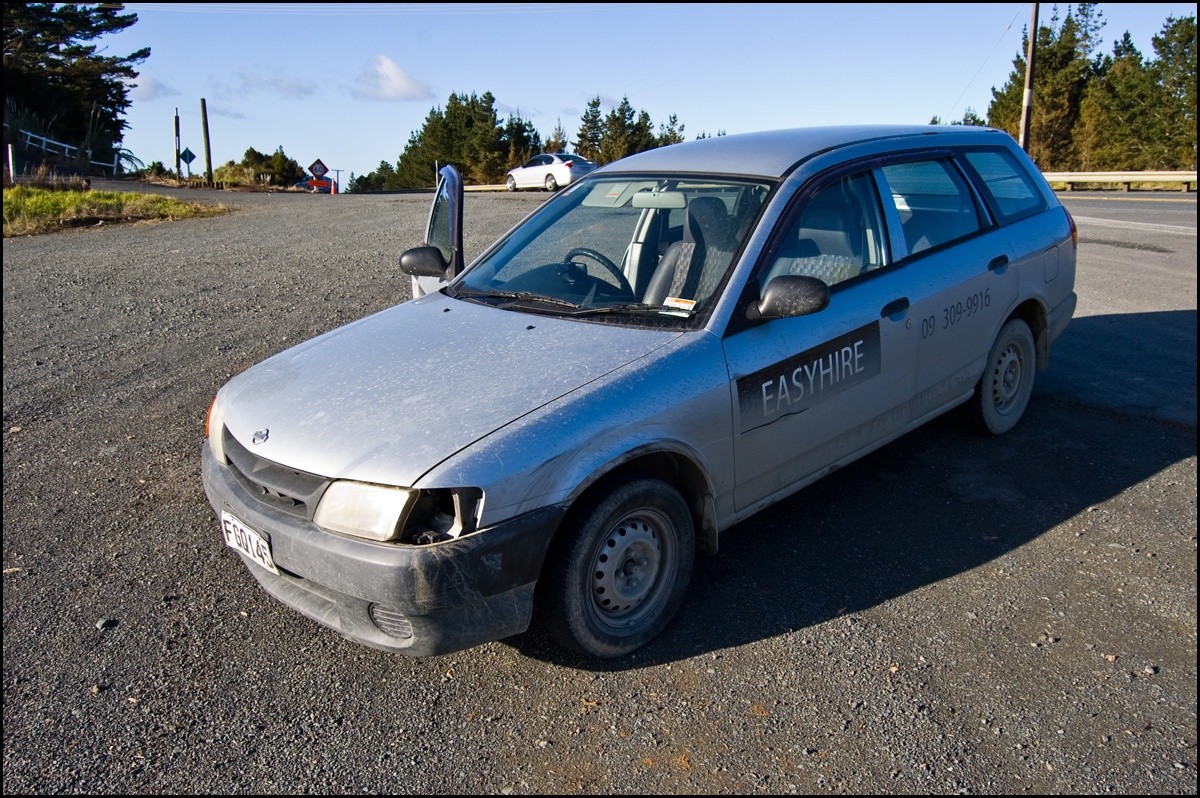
[329,177,337,195]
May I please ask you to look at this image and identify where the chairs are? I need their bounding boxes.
[642,177,970,305]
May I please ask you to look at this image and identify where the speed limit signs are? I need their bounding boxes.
[308,158,330,180]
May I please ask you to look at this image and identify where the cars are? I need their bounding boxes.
[293,175,338,194]
[505,152,600,192]
[202,121,1079,663]
